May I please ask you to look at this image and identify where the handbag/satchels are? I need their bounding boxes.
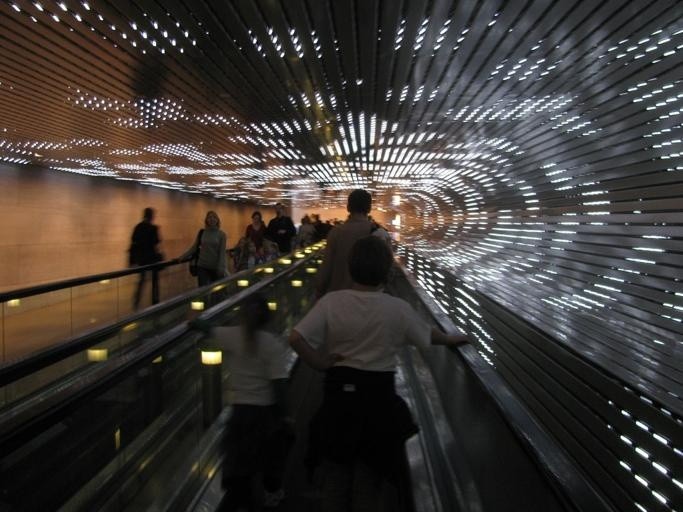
[189,229,208,277]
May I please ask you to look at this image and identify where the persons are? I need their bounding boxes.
[171,189,391,304]
[188,294,299,512]
[125,205,163,309]
[287,234,474,512]
